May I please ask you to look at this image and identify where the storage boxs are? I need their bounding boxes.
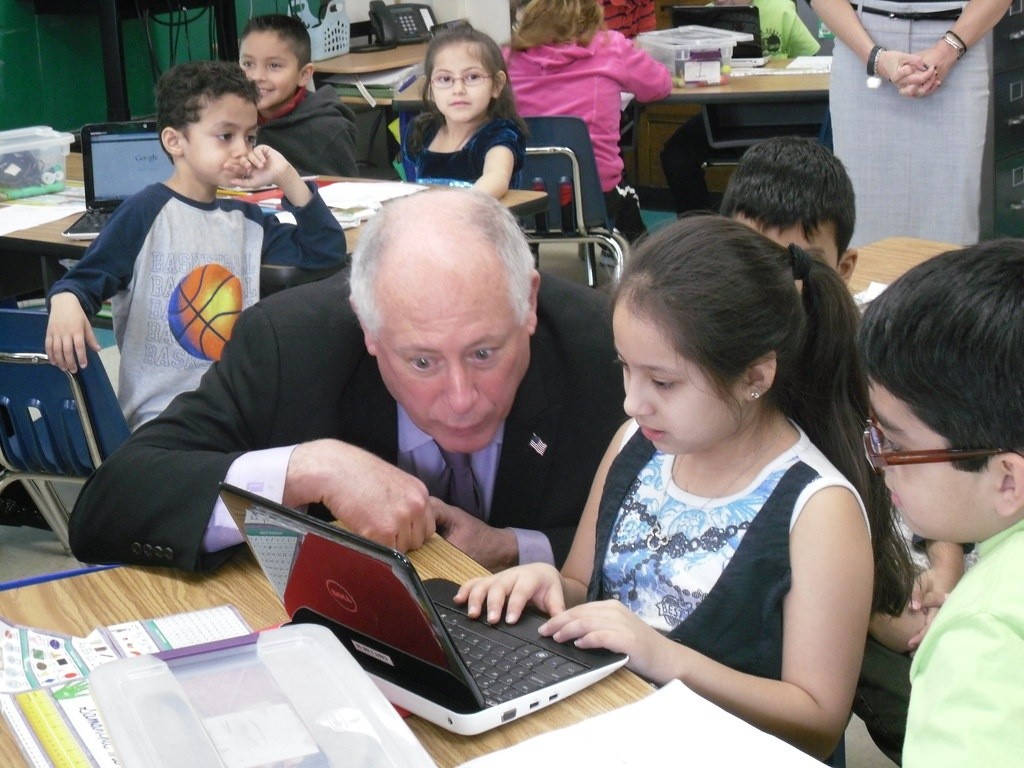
[0,126,75,202]
[639,23,754,89]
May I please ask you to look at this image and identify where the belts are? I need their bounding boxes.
[850,3,962,22]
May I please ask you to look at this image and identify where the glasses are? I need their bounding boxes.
[431,71,493,89]
[863,406,1024,477]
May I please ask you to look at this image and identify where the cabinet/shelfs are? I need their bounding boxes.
[979,0,1024,241]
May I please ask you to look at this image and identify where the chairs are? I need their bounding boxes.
[0,101,627,558]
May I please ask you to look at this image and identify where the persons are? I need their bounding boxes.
[238,14,361,177]
[498,0,821,265]
[807,0,1013,245]
[44,60,348,435]
[70,191,633,578]
[453,135,1024,768]
[392,23,531,201]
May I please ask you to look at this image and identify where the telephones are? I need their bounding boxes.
[368,0,438,45]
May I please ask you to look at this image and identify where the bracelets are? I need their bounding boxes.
[866,46,886,79]
[940,31,967,60]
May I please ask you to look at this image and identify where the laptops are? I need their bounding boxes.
[216,480,629,735]
[671,6,771,67]
[63,121,175,240]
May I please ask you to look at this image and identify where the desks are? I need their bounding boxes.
[314,41,431,106]
[0,521,659,767]
[0,152,549,349]
[633,57,832,214]
[841,236,969,317]
